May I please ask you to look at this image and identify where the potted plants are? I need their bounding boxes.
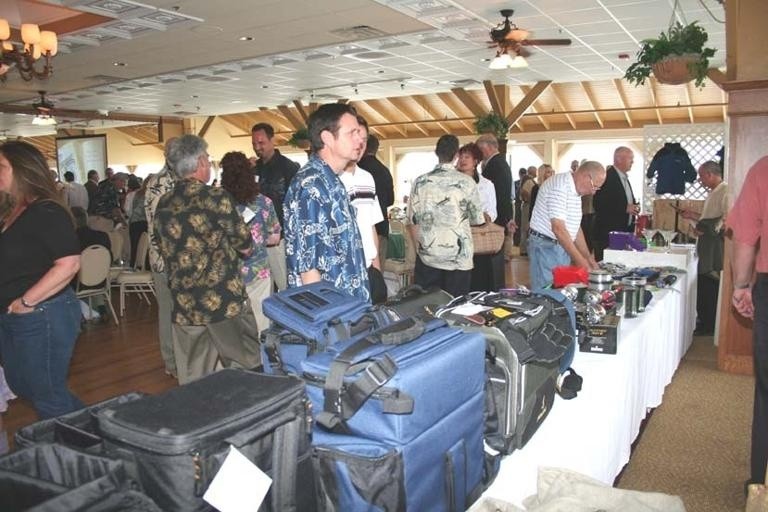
[472,110,509,162]
[286,127,312,150]
[621,20,719,92]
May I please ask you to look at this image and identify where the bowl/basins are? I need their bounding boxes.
[608,231,630,249]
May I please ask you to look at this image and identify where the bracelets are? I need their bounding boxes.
[733,283,750,289]
[19,297,34,309]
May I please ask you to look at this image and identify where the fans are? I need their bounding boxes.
[458,9,571,55]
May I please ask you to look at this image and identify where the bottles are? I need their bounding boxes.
[560,281,623,324]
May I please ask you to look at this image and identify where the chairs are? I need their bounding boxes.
[75,215,157,326]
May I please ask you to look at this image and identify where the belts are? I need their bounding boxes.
[529,229,558,243]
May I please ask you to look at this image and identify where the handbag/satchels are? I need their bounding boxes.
[471,213,506,254]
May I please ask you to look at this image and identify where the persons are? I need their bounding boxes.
[56,168,152,316]
[515,157,599,256]
[594,146,642,248]
[218,150,283,335]
[248,124,301,224]
[212,179,217,186]
[526,160,607,288]
[143,137,181,378]
[0,142,91,421]
[726,152,766,494]
[674,160,727,337]
[337,114,395,306]
[406,133,518,299]
[151,134,263,387]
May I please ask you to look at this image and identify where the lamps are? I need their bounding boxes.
[489,44,531,71]
[32,90,58,127]
[0,18,59,81]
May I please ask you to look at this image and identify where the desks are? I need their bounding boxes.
[462,237,700,512]
